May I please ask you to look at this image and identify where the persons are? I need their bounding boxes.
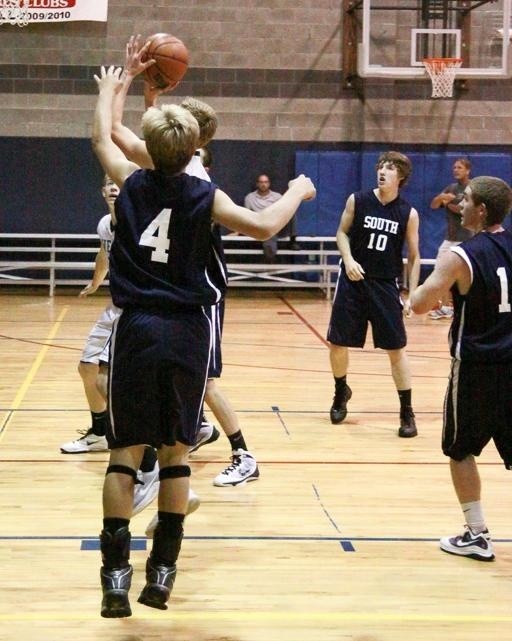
[111,31,259,536]
[407,173,512,565]
[90,67,318,619]
[227,175,301,266]
[324,147,421,439]
[424,156,476,320]
[58,171,125,458]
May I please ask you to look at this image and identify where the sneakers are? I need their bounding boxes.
[130,460,162,517]
[137,559,177,609]
[428,305,454,320]
[398,405,419,438]
[99,565,133,616]
[59,431,108,453]
[211,452,259,487]
[174,422,220,456]
[439,527,494,562]
[332,383,353,423]
[146,487,200,536]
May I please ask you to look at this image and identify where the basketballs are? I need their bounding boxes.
[140,33,190,88]
